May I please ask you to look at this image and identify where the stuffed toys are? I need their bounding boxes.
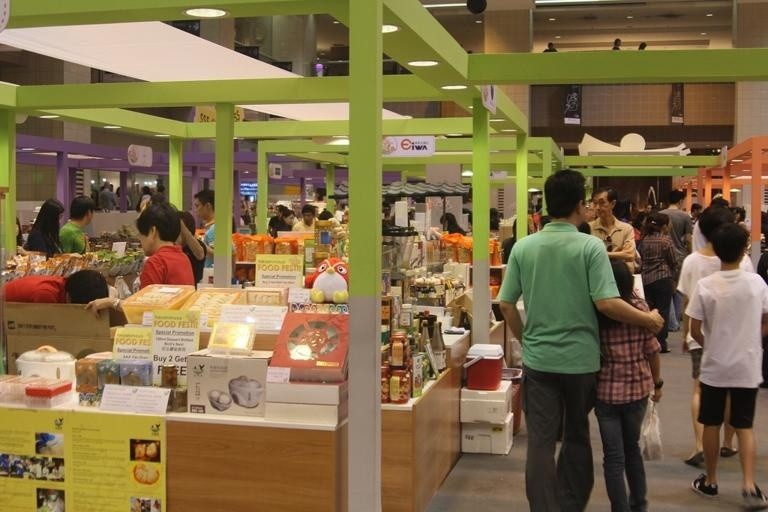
[305,258,349,304]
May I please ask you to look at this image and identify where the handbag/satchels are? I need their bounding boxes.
[639,391,662,463]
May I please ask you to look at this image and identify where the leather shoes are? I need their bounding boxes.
[720,446,739,458]
[685,451,707,465]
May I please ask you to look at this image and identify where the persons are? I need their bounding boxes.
[0,454,64,481]
[635,189,767,500]
[134,498,161,512]
[0,270,109,304]
[490,171,666,511]
[146,442,159,462]
[240,205,413,243]
[16,218,26,249]
[24,200,64,262]
[134,442,146,461]
[56,196,96,255]
[42,489,65,512]
[85,182,237,319]
[440,213,466,236]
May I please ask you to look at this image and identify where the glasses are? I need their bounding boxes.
[605,235,613,252]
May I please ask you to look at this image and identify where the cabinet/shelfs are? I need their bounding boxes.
[381,368,457,512]
[0,403,350,512]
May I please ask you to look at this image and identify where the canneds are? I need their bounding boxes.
[160,363,178,388]
[373,294,431,402]
[171,385,185,411]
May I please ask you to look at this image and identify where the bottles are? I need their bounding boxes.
[411,310,447,380]
[382,267,464,306]
[381,295,424,404]
[303,220,349,288]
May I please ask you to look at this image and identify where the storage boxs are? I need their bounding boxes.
[462,343,505,390]
[460,381,512,425]
[460,413,515,455]
[2,300,112,375]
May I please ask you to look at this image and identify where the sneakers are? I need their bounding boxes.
[690,475,718,499]
[742,484,768,509]
